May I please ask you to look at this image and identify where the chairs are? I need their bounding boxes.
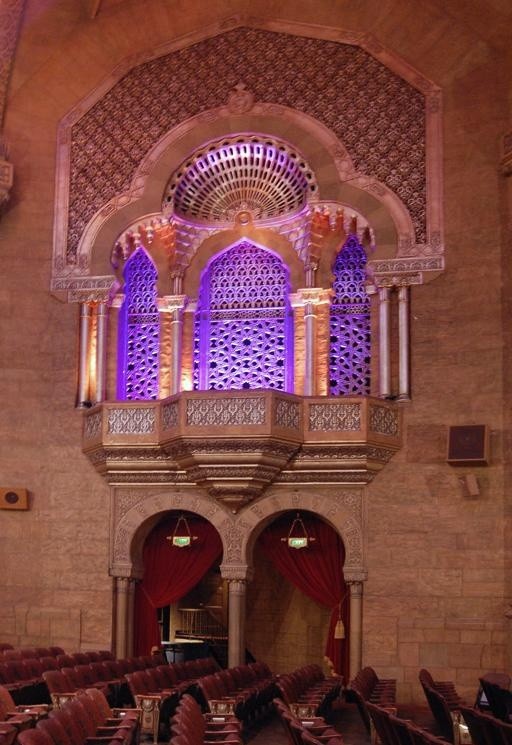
[0,643,512,745]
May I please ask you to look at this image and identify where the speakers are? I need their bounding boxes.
[0,487,28,512]
